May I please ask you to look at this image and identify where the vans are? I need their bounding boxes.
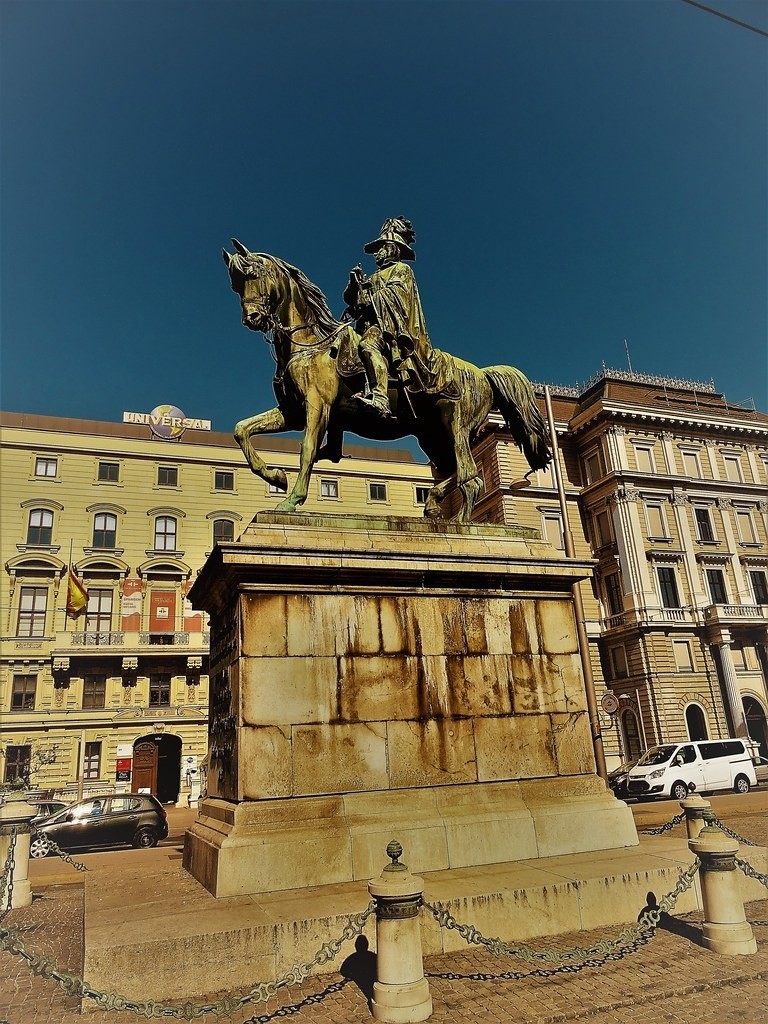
[624,738,758,799]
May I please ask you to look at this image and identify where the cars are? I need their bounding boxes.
[30,793,170,858]
[608,759,643,800]
[0,799,72,821]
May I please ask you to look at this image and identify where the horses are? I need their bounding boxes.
[215,236,553,524]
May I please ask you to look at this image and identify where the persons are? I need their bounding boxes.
[342,216,454,420]
[90,801,101,815]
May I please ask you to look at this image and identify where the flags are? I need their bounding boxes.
[68,569,91,614]
